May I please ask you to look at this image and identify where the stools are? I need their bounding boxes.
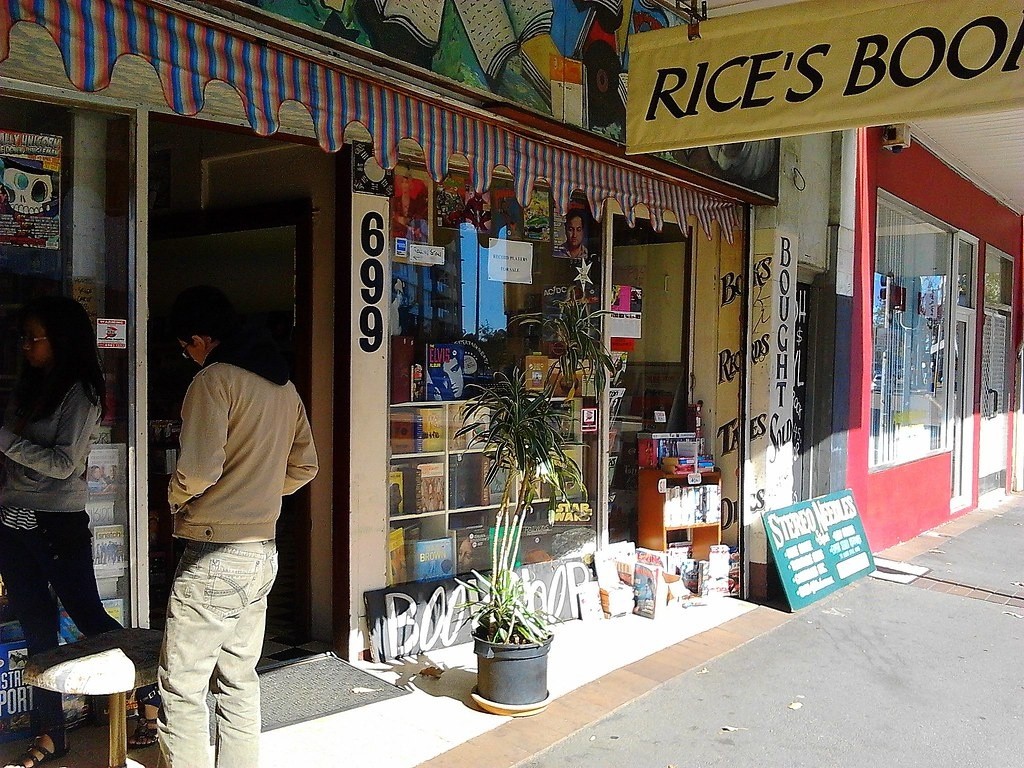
[23,627,165,768]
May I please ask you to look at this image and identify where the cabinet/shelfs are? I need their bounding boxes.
[389,398,584,540]
[637,465,723,563]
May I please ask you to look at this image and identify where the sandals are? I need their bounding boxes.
[127,684,166,749]
[3,731,70,768]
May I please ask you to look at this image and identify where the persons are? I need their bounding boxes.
[554,208,590,258]
[157,284,320,768]
[0,293,161,767]
[426,346,463,401]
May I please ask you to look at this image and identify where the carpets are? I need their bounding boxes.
[204,656,410,747]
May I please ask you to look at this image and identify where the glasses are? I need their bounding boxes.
[182,339,194,358]
[17,336,47,348]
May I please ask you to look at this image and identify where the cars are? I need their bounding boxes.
[871,369,905,437]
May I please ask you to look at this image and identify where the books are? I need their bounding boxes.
[651,432,714,474]
[410,345,465,401]
[387,500,599,580]
[668,483,721,526]
[576,539,742,620]
[389,446,583,512]
[390,404,493,449]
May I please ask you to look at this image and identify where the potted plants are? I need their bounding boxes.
[450,285,625,718]
